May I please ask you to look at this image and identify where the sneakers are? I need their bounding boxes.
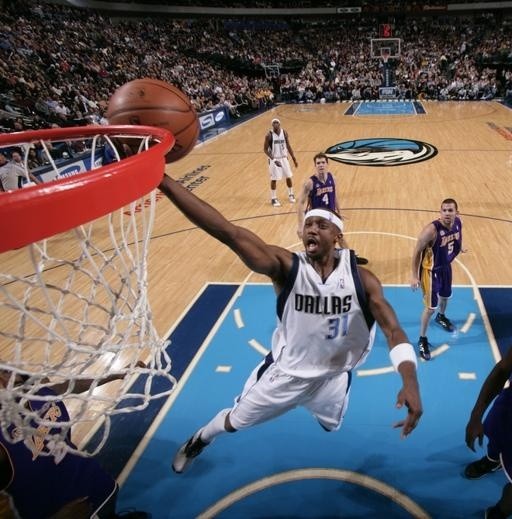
[272,198,280,206]
[289,194,296,203]
[464,455,503,480]
[418,335,431,360]
[434,312,457,333]
[172,430,210,474]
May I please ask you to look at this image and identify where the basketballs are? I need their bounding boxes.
[107,79,200,164]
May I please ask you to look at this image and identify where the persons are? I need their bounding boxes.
[297,152,368,265]
[276,1,512,103]
[411,199,468,361]
[2,1,276,194]
[1,361,153,519]
[465,347,512,517]
[263,118,298,207]
[120,143,423,475]
